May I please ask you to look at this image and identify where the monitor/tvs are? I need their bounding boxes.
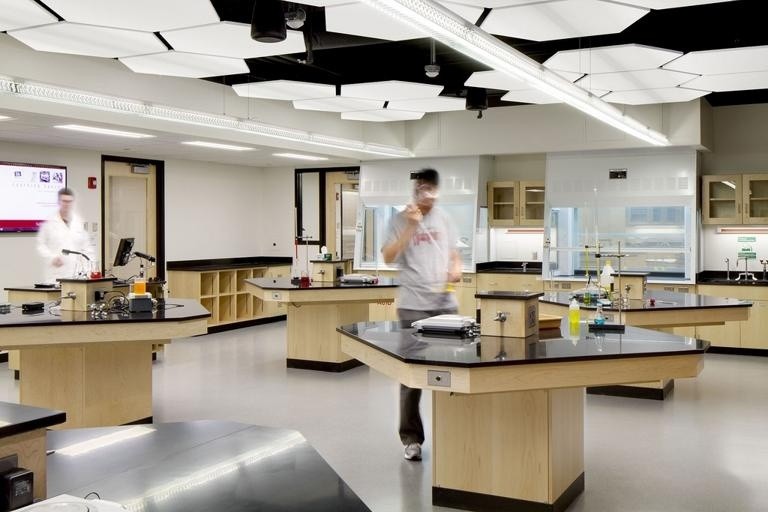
[112,237,135,267]
[0,161,68,233]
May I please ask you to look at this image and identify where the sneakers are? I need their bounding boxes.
[404,441,423,460]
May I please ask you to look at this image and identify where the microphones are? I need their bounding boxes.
[61,249,81,255]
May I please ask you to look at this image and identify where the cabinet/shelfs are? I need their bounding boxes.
[166,266,294,329]
[702,174,768,226]
[696,285,768,355]
[485,178,546,228]
[476,272,543,311]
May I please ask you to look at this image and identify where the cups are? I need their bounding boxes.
[90,261,99,278]
[584,284,591,303]
[301,271,310,288]
[134,278,146,295]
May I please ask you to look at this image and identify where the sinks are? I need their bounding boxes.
[712,279,768,283]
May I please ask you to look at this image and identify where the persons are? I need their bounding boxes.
[35,188,97,284]
[380,167,462,462]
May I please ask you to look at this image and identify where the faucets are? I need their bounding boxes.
[737,258,747,272]
[723,258,729,279]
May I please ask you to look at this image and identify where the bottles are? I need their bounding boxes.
[594,303,604,324]
[568,301,580,338]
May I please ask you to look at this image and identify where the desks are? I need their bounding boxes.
[244,275,402,374]
[336,316,711,512]
[43,419,373,512]
[534,288,754,401]
[3,280,165,379]
[0,298,212,429]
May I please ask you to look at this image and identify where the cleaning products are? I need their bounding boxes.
[568,297,580,323]
[569,323,581,347]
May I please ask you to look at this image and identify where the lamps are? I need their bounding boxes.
[4,74,413,159]
[425,36,440,79]
[286,3,306,30]
[366,0,671,149]
